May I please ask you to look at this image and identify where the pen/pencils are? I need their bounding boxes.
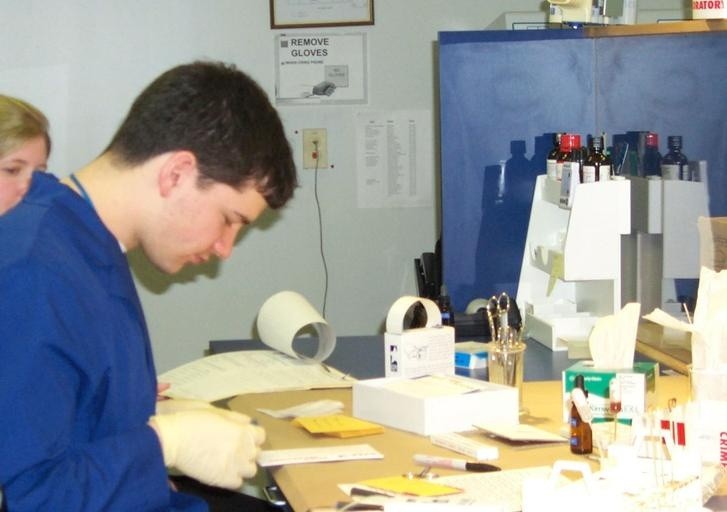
[413,454,501,471]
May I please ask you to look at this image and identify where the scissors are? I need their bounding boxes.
[486,292,518,386]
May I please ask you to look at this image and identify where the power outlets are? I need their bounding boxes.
[303,128,327,170]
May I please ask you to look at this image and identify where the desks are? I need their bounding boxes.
[211,334,727,511]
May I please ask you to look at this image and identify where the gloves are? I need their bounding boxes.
[149,395,267,492]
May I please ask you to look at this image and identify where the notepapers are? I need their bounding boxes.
[290,414,384,438]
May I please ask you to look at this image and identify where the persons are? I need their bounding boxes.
[0,57,305,511]
[0,89,55,219]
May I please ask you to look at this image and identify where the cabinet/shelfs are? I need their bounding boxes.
[622,161,713,339]
[514,176,632,353]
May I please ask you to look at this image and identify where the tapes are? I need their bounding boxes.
[464,298,495,315]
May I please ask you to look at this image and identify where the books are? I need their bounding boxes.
[153,291,365,406]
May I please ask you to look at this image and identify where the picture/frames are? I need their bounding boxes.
[270,0,375,29]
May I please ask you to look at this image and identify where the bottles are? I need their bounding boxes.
[546,131,612,209]
[568,374,594,456]
[660,135,690,181]
[643,132,663,177]
